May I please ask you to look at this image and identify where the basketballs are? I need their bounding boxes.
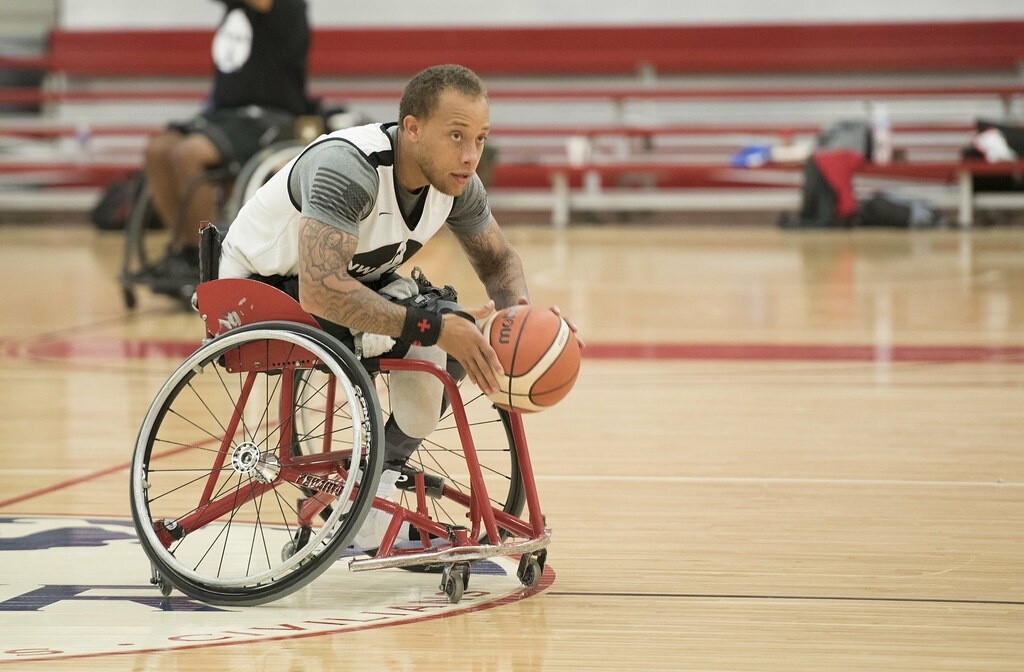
[477,304,581,414]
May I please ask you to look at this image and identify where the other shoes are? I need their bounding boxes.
[152,244,200,297]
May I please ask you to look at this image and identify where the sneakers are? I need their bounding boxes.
[331,467,465,548]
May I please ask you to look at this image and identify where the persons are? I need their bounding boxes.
[121,0,309,292]
[218,64,584,549]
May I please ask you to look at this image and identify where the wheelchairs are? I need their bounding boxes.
[127,216,548,608]
[118,96,347,311]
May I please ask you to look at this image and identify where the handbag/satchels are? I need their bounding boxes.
[92,171,163,228]
[802,152,861,227]
[861,197,937,228]
[823,121,872,159]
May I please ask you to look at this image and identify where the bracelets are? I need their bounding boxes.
[391,305,442,347]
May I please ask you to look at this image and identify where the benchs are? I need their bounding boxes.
[0,23,1024,228]
